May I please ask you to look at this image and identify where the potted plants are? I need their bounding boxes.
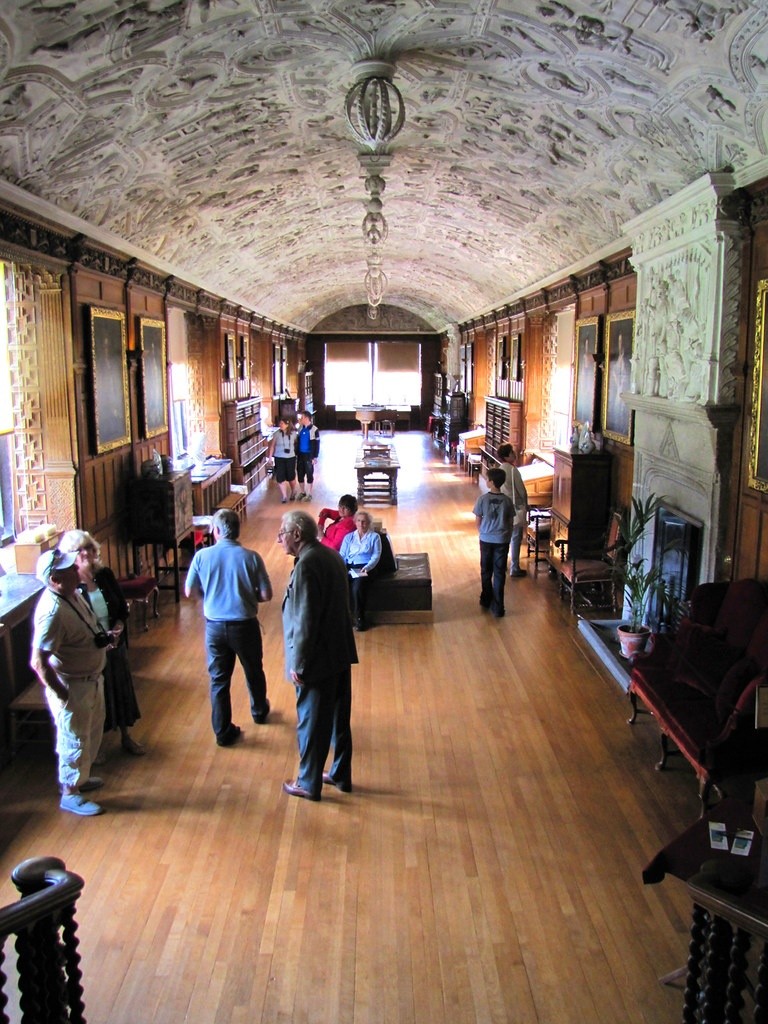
[617,493,690,661]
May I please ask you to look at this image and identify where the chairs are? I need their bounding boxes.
[555,506,627,615]
[526,504,552,566]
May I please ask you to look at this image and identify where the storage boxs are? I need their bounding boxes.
[15,529,64,575]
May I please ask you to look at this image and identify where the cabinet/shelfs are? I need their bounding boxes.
[549,451,613,573]
[428,372,521,475]
[130,370,316,602]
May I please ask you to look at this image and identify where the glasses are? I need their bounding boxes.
[277,530,293,540]
[50,549,60,575]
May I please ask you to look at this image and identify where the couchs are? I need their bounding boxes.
[628,577,768,821]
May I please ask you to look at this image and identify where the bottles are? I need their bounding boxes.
[579,432,596,455]
[569,426,580,447]
[160,454,174,475]
[140,459,159,480]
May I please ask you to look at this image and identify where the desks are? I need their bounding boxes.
[354,444,400,506]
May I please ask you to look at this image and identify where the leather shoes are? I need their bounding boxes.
[322,770,352,792]
[283,778,321,801]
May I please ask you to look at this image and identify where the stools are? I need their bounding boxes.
[9,676,50,759]
[117,578,159,631]
[351,553,432,626]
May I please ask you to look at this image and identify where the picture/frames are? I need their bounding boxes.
[225,334,250,379]
[458,341,474,395]
[600,308,637,445]
[510,333,521,380]
[86,305,132,453]
[498,336,506,379]
[573,316,601,430]
[748,278,768,494]
[272,344,289,396]
[135,316,169,440]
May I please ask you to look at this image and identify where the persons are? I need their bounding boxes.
[182,509,273,747]
[496,443,530,577]
[276,509,359,800]
[59,530,145,755]
[339,511,383,632]
[471,468,518,616]
[294,410,320,503]
[268,418,298,504]
[316,495,360,553]
[27,550,109,814]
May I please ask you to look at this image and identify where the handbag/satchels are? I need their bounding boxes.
[513,510,525,526]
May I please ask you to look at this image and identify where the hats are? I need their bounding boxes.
[36,549,79,586]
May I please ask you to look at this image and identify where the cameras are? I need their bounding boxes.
[95,632,115,648]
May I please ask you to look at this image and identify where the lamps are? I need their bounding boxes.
[344,58,406,322]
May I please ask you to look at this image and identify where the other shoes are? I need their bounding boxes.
[93,745,107,766]
[218,726,240,746]
[254,699,270,723]
[122,734,145,755]
[281,496,287,502]
[351,615,368,630]
[304,495,312,502]
[290,491,297,500]
[511,569,526,577]
[297,492,306,500]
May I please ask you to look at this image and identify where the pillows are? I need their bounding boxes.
[674,619,760,726]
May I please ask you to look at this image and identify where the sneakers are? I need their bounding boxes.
[60,791,103,815]
[58,777,104,794]
